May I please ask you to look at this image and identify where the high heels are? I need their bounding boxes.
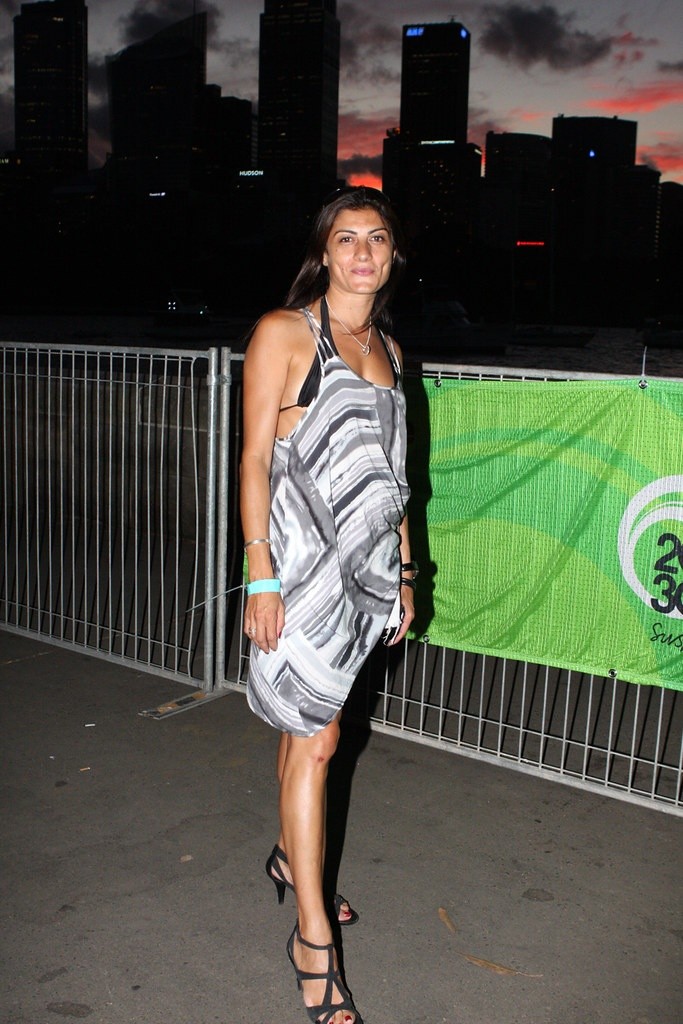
[263,844,359,926]
[285,918,364,1024]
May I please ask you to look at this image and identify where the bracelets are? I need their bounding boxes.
[400,577,417,591]
[245,578,281,596]
[243,538,275,552]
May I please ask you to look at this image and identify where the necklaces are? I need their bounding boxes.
[324,294,372,356]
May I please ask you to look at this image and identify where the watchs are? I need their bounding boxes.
[401,561,419,578]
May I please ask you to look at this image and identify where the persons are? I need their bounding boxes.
[237,186,423,1022]
